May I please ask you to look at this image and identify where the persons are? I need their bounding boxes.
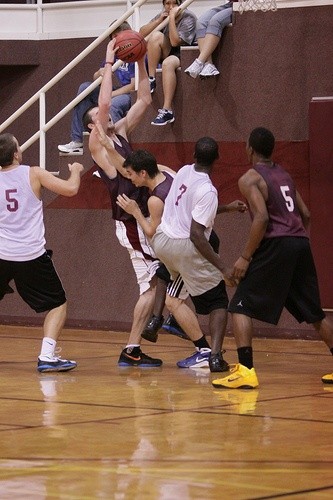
[212,127,333,388]
[0,134,84,372]
[58,21,148,155]
[94,120,220,368]
[184,0,238,78]
[142,137,247,370]
[139,0,198,126]
[82,37,188,368]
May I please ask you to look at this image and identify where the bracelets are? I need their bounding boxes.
[105,62,114,66]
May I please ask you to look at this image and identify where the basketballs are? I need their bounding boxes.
[114,29,148,64]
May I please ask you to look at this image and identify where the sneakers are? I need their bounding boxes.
[118,346,163,368]
[213,387,257,413]
[176,346,211,368]
[151,108,176,126]
[37,356,77,373]
[321,373,333,383]
[141,315,164,343]
[58,141,84,153]
[147,77,157,93]
[209,356,229,372]
[212,363,259,388]
[184,58,204,79]
[199,64,220,79]
[162,313,191,340]
[322,384,333,392]
